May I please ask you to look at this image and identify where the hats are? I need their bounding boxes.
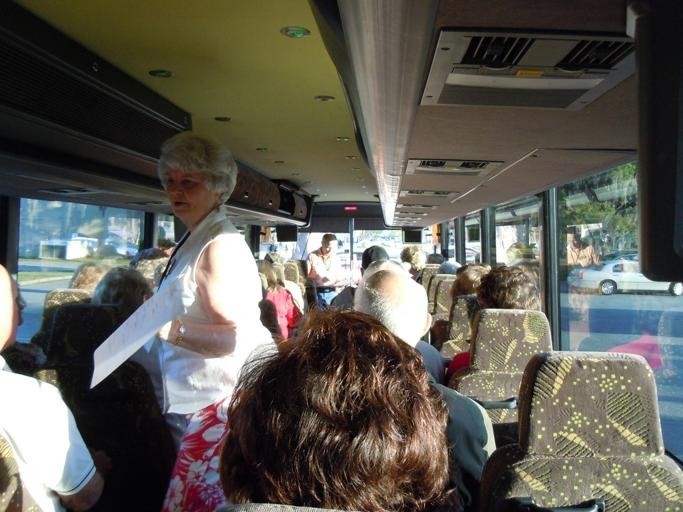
[362,246,389,267]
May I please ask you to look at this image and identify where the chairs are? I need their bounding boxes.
[447,307,553,449]
[477,348,683,512]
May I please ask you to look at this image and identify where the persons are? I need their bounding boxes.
[606,310,665,368]
[567,279,611,352]
[214,308,472,512]
[353,270,498,512]
[400,243,542,386]
[94,245,175,303]
[259,229,402,342]
[565,226,601,267]
[150,130,263,512]
[0,263,112,512]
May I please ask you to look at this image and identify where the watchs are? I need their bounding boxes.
[174,318,188,346]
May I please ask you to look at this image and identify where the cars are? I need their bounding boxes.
[568,259,683,297]
[90,229,143,257]
[599,252,638,261]
[443,245,480,265]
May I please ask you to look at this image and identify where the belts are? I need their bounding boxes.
[318,288,336,293]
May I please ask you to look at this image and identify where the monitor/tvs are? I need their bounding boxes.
[276,225,297,242]
[402,229,423,244]
